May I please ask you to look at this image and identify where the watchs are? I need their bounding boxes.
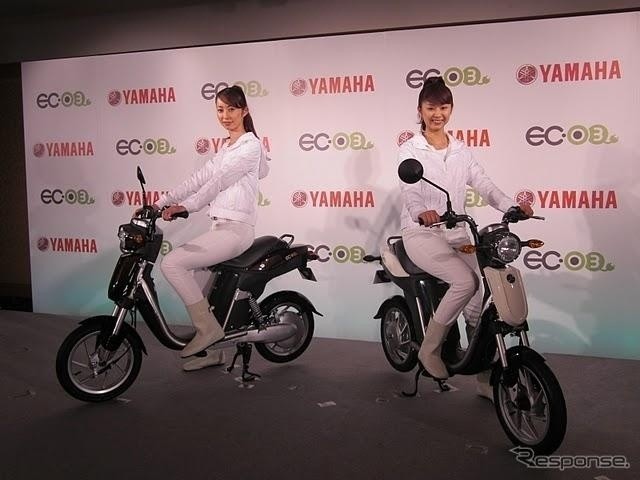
[56,168,323,404]
[363,159,567,455]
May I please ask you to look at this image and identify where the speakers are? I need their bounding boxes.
[417,317,450,381]
[183,348,226,371]
[476,370,494,404]
[180,297,224,358]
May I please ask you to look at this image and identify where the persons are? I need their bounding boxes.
[132,85,263,372]
[394,75,534,381]
